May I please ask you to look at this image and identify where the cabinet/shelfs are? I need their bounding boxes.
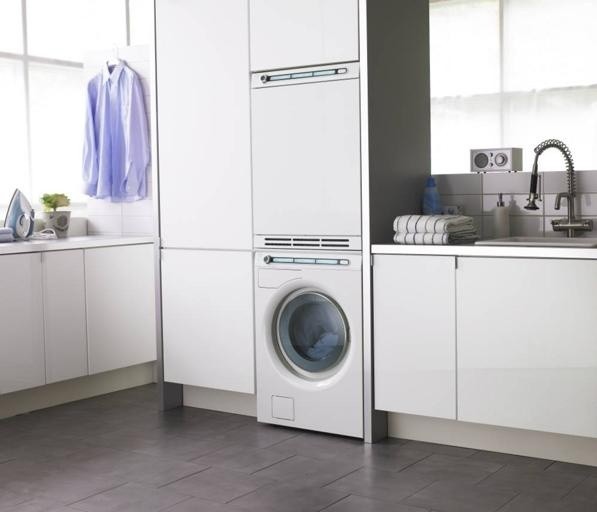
[252,79,430,235]
[250,0,430,75]
[42,242,159,410]
[372,252,457,446]
[159,248,258,420]
[154,1,253,250]
[456,258,596,470]
[0,245,46,420]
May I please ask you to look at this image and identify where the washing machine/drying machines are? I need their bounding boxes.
[253,250,364,440]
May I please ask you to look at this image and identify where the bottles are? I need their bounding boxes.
[493,201,511,237]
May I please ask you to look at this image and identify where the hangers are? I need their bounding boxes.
[105,45,120,67]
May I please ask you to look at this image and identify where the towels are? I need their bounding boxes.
[393,214,480,245]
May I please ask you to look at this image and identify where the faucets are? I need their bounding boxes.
[523,138,575,222]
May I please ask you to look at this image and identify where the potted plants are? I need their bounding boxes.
[40,193,71,238]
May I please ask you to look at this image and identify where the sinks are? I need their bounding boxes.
[474,236,597,248]
